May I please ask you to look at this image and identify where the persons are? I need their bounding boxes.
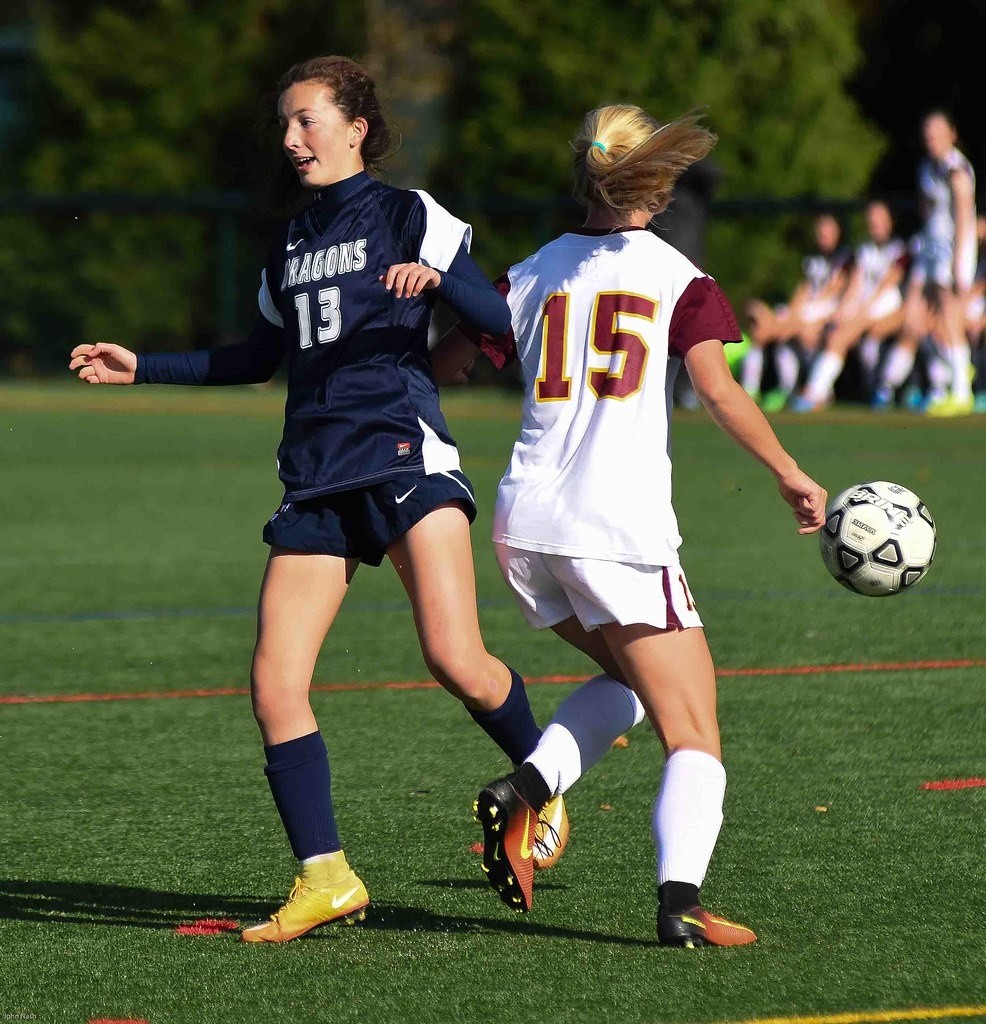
[744,109,986,417]
[69,57,569,942]
[428,104,828,948]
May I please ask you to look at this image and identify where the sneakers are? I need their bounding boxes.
[658,906,757,953]
[241,850,370,945]
[530,785,569,871]
[472,777,561,916]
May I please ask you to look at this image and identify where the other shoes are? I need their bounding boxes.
[745,387,975,422]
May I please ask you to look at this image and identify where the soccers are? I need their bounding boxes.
[820,481,938,598]
